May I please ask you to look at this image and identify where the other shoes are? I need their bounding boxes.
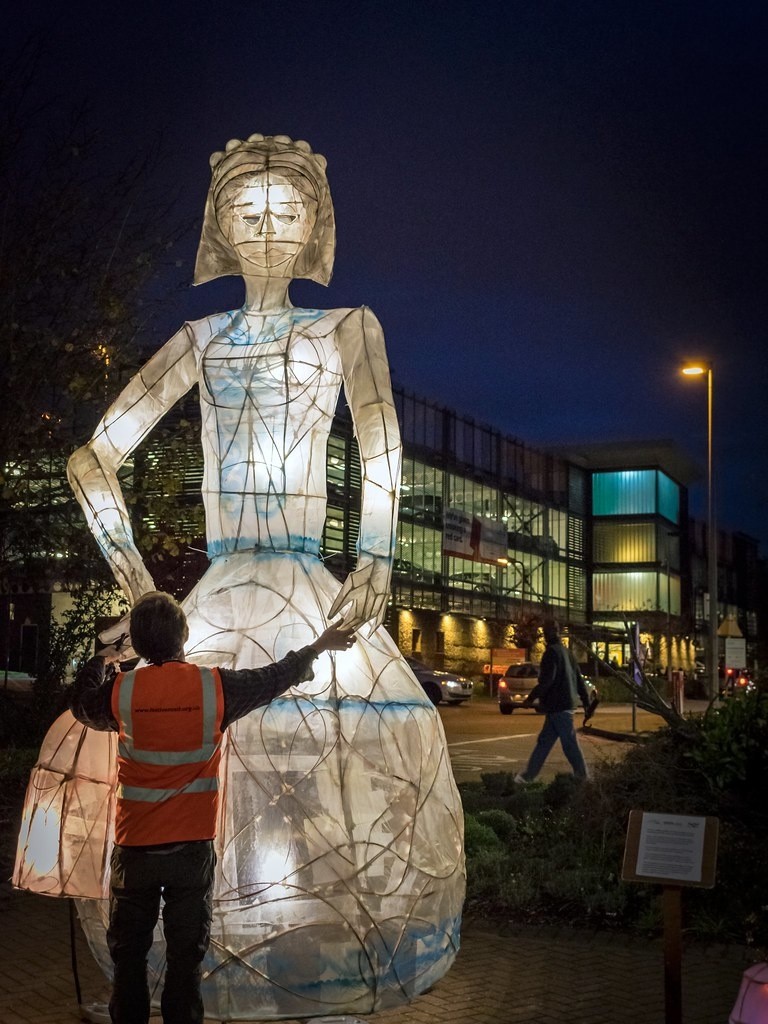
[521,772,534,782]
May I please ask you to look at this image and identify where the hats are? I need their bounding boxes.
[543,619,561,638]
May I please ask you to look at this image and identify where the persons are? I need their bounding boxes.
[70,589,359,1024]
[514,618,594,783]
[12,133,469,1024]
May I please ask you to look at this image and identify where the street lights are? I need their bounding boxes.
[496,556,525,621]
[682,365,720,703]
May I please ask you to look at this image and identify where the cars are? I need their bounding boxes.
[397,494,442,520]
[404,655,474,707]
[449,571,498,593]
[498,661,598,714]
[391,557,440,583]
